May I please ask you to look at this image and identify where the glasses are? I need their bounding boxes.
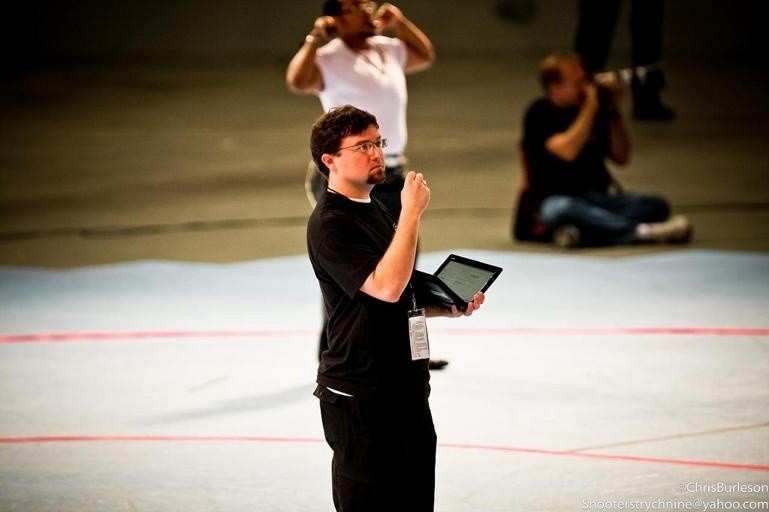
[338,3,377,15]
[335,138,388,153]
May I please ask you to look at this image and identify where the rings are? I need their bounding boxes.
[421,180,426,185]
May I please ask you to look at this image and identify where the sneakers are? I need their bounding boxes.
[555,227,582,246]
[649,215,691,242]
[428,360,448,368]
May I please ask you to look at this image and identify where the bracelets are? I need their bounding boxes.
[304,33,323,45]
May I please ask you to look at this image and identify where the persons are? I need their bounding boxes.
[575,1,679,123]
[304,103,485,512]
[517,49,696,252]
[283,0,452,371]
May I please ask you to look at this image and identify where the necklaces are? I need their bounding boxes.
[345,42,388,75]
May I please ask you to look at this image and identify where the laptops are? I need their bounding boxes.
[413,254,503,311]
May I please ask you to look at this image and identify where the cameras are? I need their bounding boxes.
[586,65,650,98]
